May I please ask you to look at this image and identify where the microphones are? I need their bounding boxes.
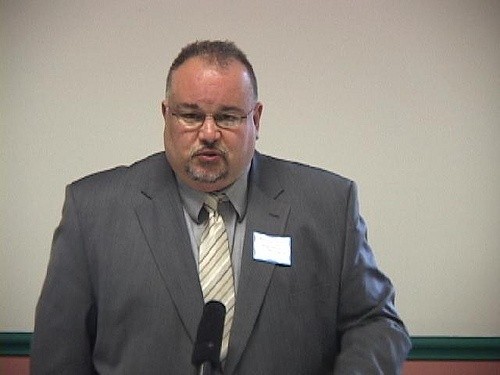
[191,301,226,375]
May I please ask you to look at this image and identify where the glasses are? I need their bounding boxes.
[168,104,255,130]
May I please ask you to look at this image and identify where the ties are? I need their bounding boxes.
[198,193,237,373]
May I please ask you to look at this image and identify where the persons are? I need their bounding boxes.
[29,39,414,375]
